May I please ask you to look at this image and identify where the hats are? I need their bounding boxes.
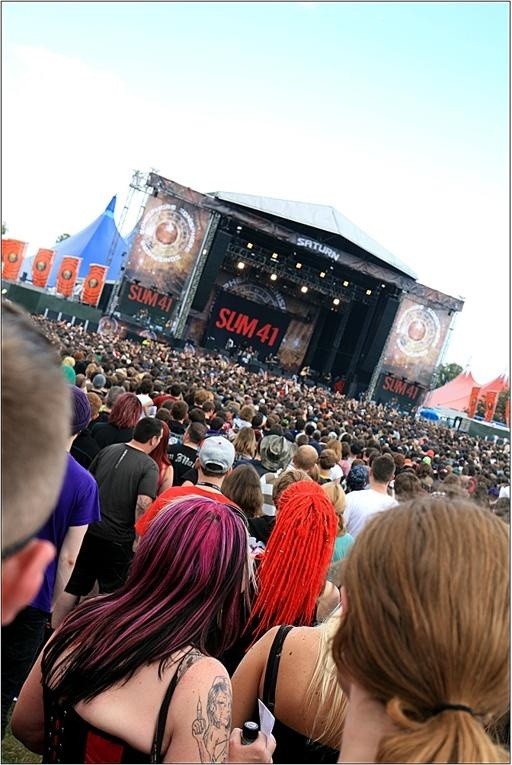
[259,434,294,470]
[62,364,74,385]
[345,466,369,488]
[93,375,105,389]
[199,436,235,473]
[70,382,92,433]
[64,356,75,366]
